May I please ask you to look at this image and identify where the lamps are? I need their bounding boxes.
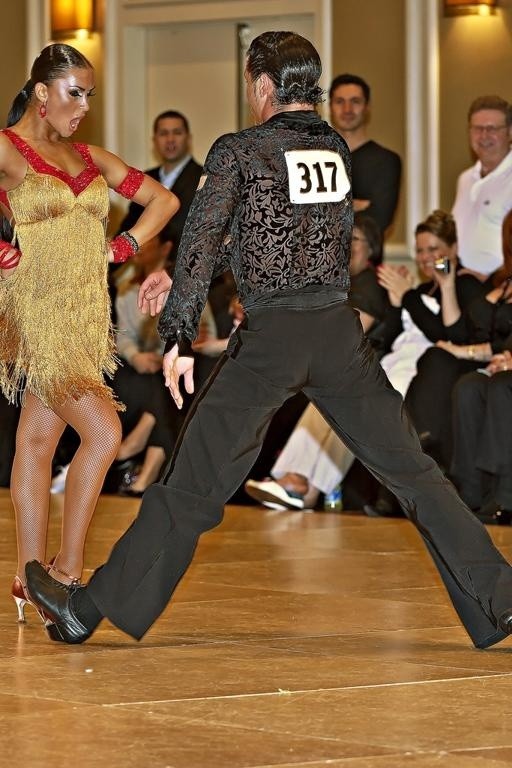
[446,0,495,16]
[51,0,103,40]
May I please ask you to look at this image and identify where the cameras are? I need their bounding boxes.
[434,257,449,274]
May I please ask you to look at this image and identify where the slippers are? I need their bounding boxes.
[245,479,304,510]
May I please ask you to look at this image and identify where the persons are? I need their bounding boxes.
[1,43,181,622]
[25,31,512,649]
[115,110,206,264]
[230,207,511,524]
[327,74,403,263]
[449,94,512,274]
[46,226,241,497]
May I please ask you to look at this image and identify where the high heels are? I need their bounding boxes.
[10,555,91,643]
